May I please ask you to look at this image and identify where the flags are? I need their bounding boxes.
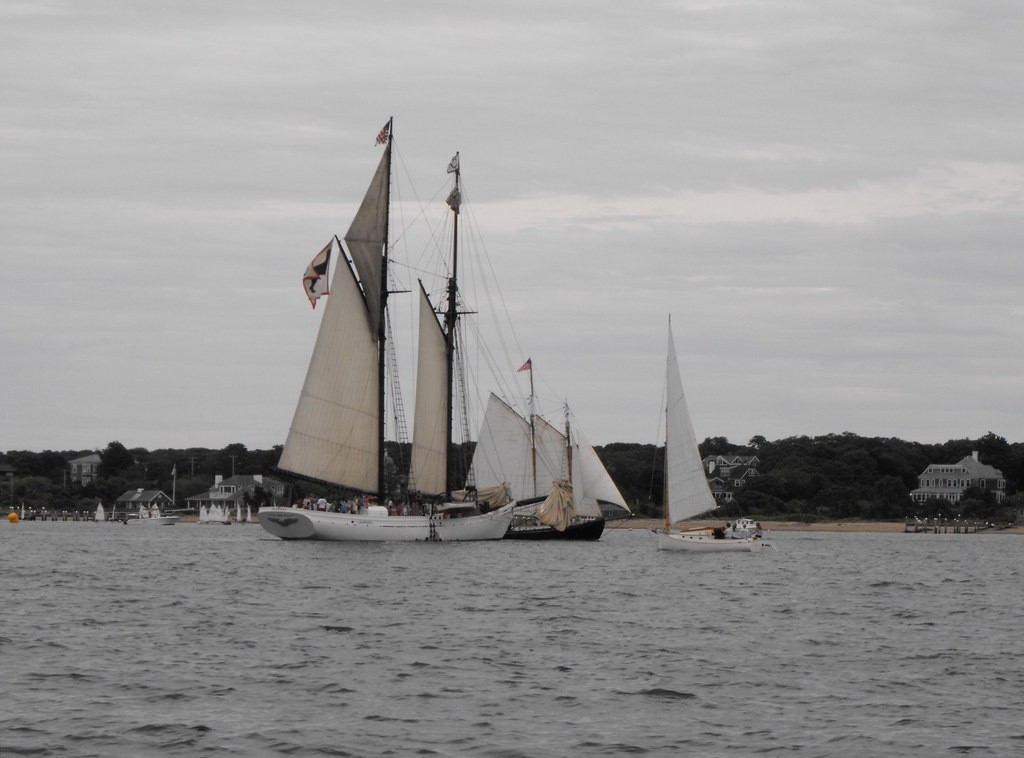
[518,358,531,371]
[303,237,334,309]
[375,119,391,146]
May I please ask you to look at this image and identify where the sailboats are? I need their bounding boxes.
[94,502,106,521]
[640,313,774,555]
[196,504,259,526]
[252,116,550,543]
[137,504,150,519]
[149,502,160,518]
[448,353,638,542]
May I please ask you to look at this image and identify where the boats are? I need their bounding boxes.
[122,515,181,527]
[734,516,757,533]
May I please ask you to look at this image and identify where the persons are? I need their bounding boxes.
[752,523,763,538]
[293,493,327,511]
[415,490,425,516]
[388,499,403,516]
[340,495,369,515]
[723,523,736,538]
[741,524,748,537]
